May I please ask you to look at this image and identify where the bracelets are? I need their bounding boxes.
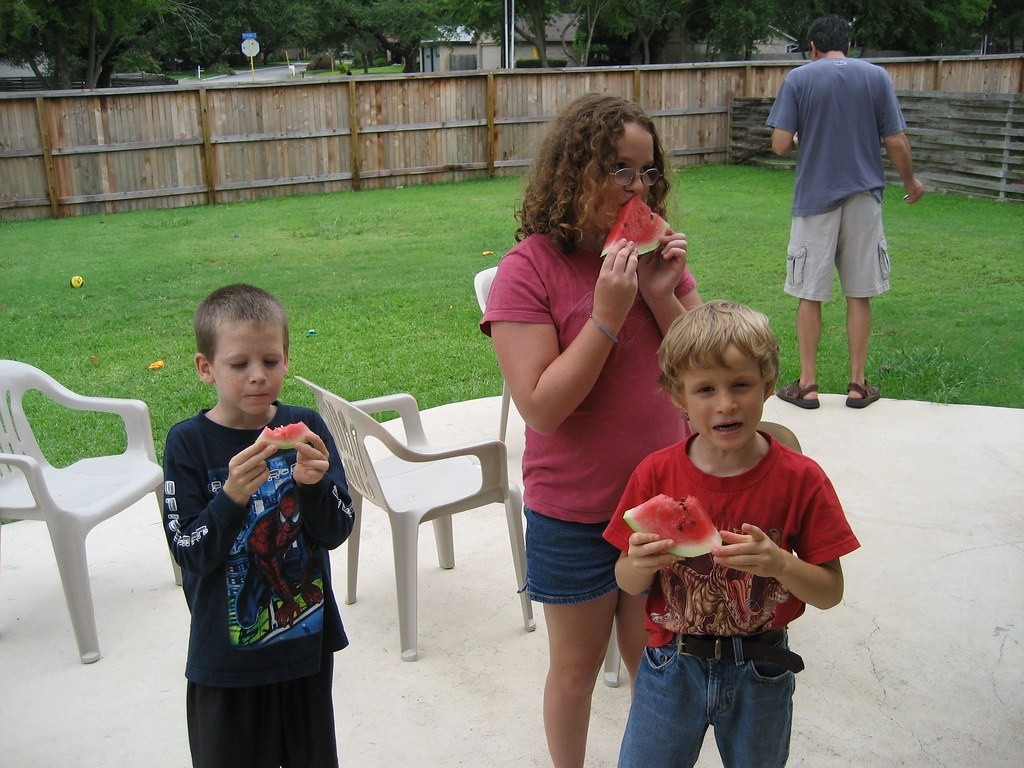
[589,313,618,342]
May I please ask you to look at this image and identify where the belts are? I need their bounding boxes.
[672,631,804,674]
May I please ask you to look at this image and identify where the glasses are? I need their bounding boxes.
[602,167,663,186]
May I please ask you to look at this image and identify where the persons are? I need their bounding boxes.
[480,93,701,768]
[163,284,355,768]
[603,299,860,768]
[766,15,923,410]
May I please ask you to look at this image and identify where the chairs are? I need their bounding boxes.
[0,360,183,662]
[293,374,536,661]
[472,267,512,445]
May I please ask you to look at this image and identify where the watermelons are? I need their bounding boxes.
[622,493,722,557]
[255,422,316,449]
[599,195,672,259]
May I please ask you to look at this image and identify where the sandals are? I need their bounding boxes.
[777,379,820,409]
[845,378,880,409]
